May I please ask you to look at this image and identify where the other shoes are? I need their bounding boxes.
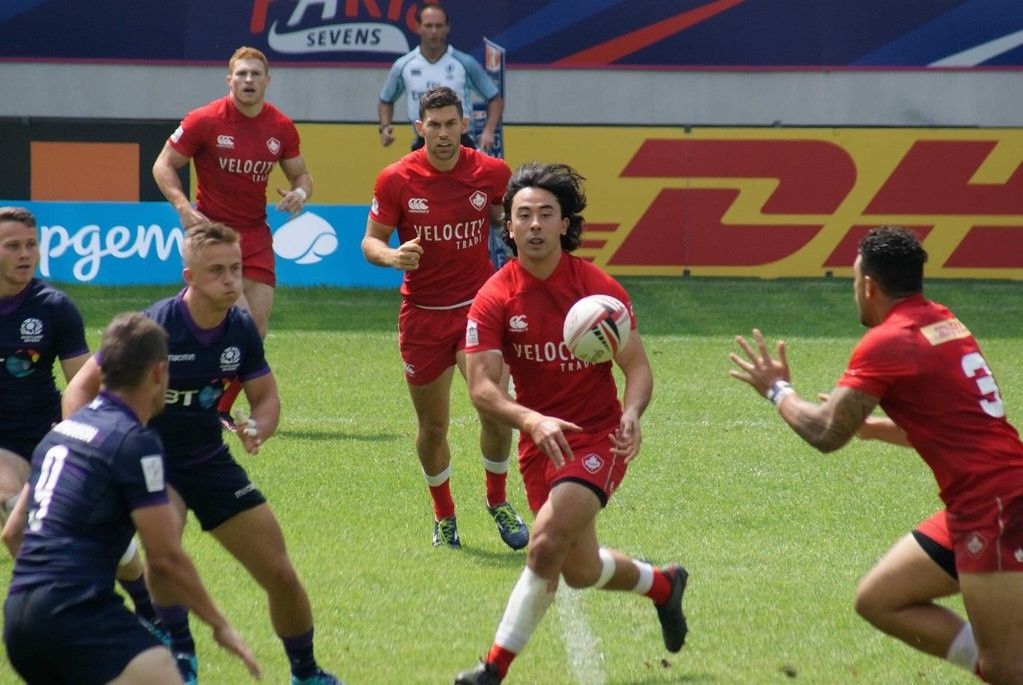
[220,412,237,432]
[176,654,197,685]
[137,614,171,647]
[291,666,345,685]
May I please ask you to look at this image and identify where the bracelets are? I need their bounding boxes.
[293,185,307,202]
[767,379,796,409]
[379,123,389,133]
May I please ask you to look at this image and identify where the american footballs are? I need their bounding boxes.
[562,295,631,363]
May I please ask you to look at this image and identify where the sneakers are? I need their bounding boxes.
[454,651,502,685]
[433,511,461,549]
[485,497,529,549]
[653,564,688,652]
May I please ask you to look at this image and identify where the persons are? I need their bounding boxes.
[153,45,313,432]
[361,86,529,550]
[454,160,689,685]
[0,206,340,685]
[729,226,1023,685]
[378,3,504,157]
